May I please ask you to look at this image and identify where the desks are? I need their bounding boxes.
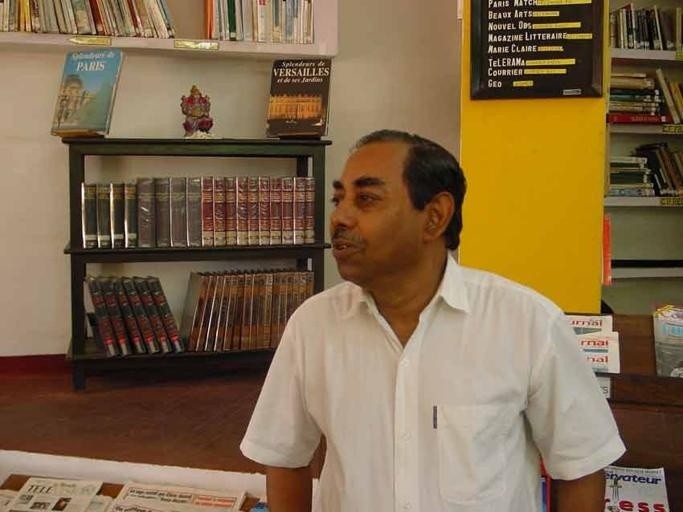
[545,313,683,512]
[0,449,318,512]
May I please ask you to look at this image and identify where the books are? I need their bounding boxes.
[607,67,683,123]
[608,142,683,197]
[83,268,315,358]
[1,0,177,39]
[50,48,129,137]
[265,57,332,141]
[202,0,313,45]
[609,2,683,50]
[82,175,316,250]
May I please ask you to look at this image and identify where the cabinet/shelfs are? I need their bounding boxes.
[62,136,332,391]
[605,0,683,281]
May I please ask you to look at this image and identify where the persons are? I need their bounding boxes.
[240,129,627,512]
